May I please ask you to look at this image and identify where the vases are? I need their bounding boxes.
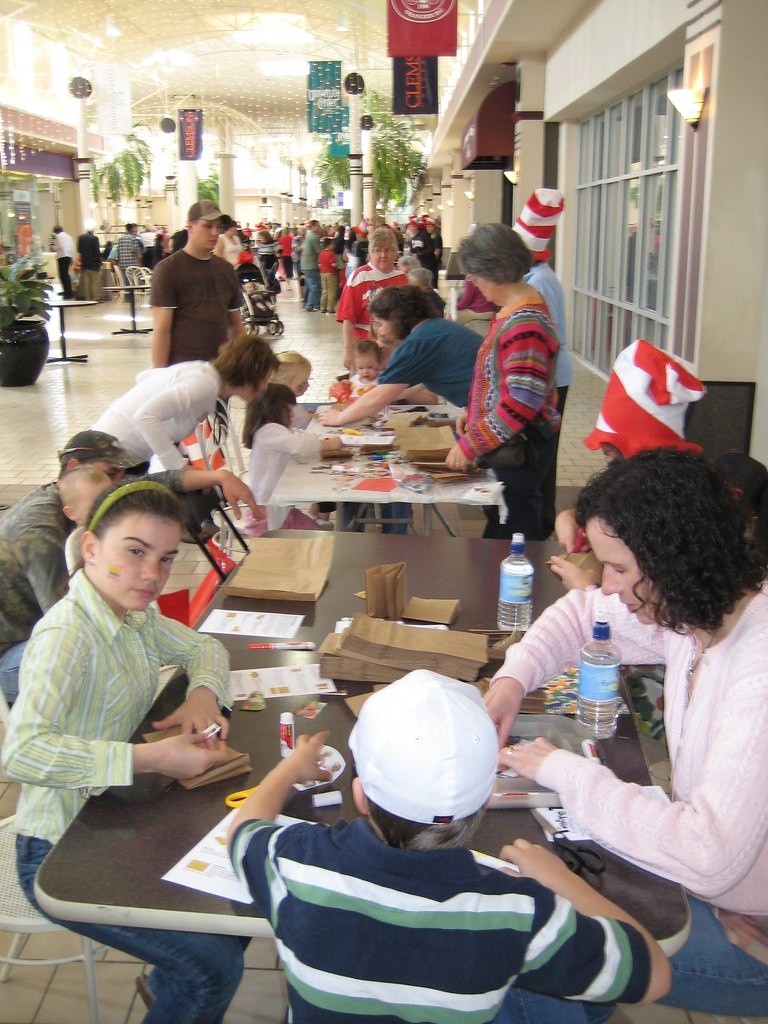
[0,320,50,386]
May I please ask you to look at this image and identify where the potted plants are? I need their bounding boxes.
[0,256,52,325]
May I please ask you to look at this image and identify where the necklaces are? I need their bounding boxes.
[693,626,720,671]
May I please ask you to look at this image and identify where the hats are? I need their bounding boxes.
[510,186,564,264]
[61,428,149,476]
[348,670,497,825]
[583,340,707,459]
[188,199,232,227]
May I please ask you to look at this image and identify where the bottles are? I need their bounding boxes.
[497,532,534,633]
[576,619,622,740]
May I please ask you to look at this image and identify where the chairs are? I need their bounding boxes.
[196,397,269,563]
[0,815,110,1024]
[113,265,153,307]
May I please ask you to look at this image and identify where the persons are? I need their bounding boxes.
[447,187,573,541]
[59,465,113,572]
[0,430,258,703]
[151,200,245,369]
[268,349,310,429]
[484,442,768,1024]
[244,384,349,537]
[217,216,370,315]
[336,228,407,370]
[50,225,75,299]
[350,341,406,405]
[97,335,279,473]
[101,223,188,301]
[77,228,101,301]
[388,214,446,313]
[0,480,251,1023]
[343,316,438,535]
[228,668,670,1023]
[550,451,768,588]
[318,285,484,425]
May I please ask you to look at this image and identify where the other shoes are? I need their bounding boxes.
[300,297,337,317]
[137,972,157,1013]
[180,521,219,542]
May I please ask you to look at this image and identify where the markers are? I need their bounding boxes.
[249,642,317,649]
[376,451,400,455]
[572,528,591,554]
[372,455,396,460]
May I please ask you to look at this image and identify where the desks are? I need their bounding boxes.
[102,286,153,334]
[34,529,691,959]
[47,301,100,363]
[274,406,502,538]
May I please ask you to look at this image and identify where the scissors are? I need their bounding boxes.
[225,786,258,807]
[530,808,606,876]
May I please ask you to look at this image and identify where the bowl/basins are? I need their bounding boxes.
[426,410,461,435]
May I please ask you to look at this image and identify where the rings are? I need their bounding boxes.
[507,747,513,757]
